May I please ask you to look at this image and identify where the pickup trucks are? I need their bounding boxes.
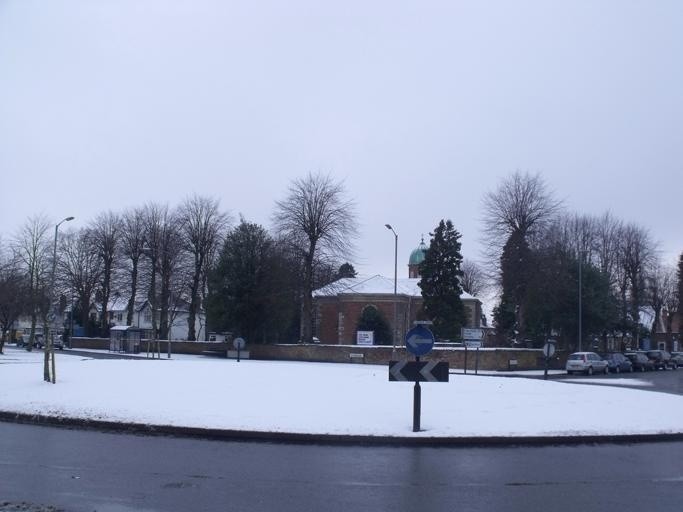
[17,325,65,349]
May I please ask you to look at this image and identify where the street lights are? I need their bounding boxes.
[384,223,398,365]
[41,216,75,382]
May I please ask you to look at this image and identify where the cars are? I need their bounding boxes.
[564,348,683,375]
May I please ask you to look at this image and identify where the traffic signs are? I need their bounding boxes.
[459,327,485,348]
[387,324,450,383]
